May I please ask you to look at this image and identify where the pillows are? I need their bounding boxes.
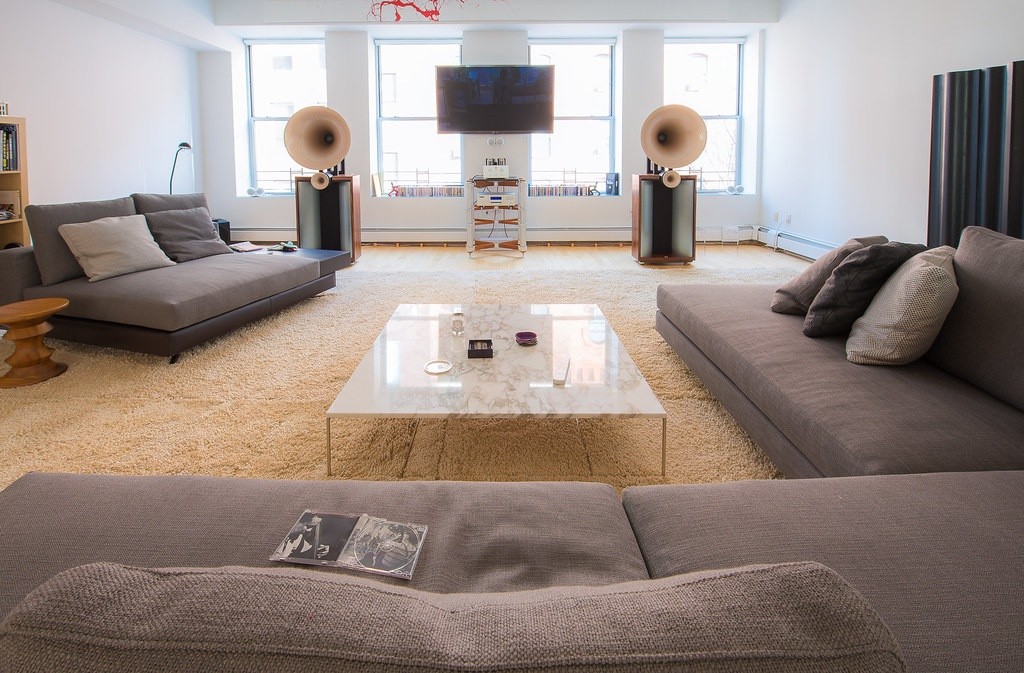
[845,245,959,365]
[803,241,926,338]
[770,234,888,314]
[24,191,234,284]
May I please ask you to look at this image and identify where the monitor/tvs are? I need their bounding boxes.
[435,65,554,134]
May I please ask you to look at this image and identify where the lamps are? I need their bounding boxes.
[247,187,265,197]
[169,142,191,196]
[487,137,504,146]
[726,185,744,195]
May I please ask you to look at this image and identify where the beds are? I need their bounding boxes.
[0,244,353,364]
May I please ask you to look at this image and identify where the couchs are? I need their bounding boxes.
[0,228,1024,673]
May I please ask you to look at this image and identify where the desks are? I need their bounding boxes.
[0,298,70,388]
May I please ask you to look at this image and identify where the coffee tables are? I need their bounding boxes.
[326,302,667,478]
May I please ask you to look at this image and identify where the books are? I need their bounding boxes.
[0,130,17,171]
[230,241,263,252]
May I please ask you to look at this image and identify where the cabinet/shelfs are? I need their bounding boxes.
[465,176,528,255]
[0,116,32,251]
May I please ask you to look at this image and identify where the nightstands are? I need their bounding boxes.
[212,218,232,243]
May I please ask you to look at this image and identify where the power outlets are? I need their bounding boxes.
[785,214,790,223]
[772,212,779,221]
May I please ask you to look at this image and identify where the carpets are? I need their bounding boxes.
[0,266,803,487]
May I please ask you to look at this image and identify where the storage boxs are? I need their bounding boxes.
[468,338,493,359]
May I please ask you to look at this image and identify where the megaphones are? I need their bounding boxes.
[663,170,680,188]
[283,105,351,170]
[640,104,707,168]
[311,172,329,190]
